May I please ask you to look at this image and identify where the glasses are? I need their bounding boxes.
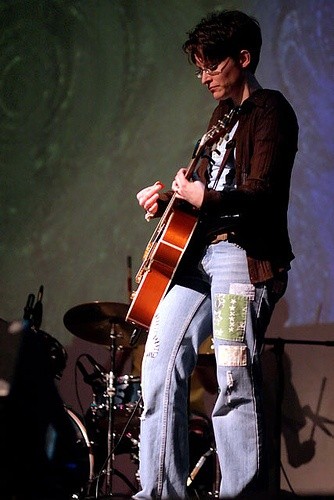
[195,57,231,79]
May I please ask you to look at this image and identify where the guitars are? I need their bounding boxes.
[125,105,240,329]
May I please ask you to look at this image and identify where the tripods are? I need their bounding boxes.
[69,321,139,500]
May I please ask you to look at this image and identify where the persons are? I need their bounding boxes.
[129,10,300,500]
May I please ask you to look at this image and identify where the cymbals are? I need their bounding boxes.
[62,302,146,349]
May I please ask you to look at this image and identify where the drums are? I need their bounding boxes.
[90,376,141,409]
[44,403,104,500]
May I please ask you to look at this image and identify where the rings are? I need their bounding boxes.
[147,210,152,214]
